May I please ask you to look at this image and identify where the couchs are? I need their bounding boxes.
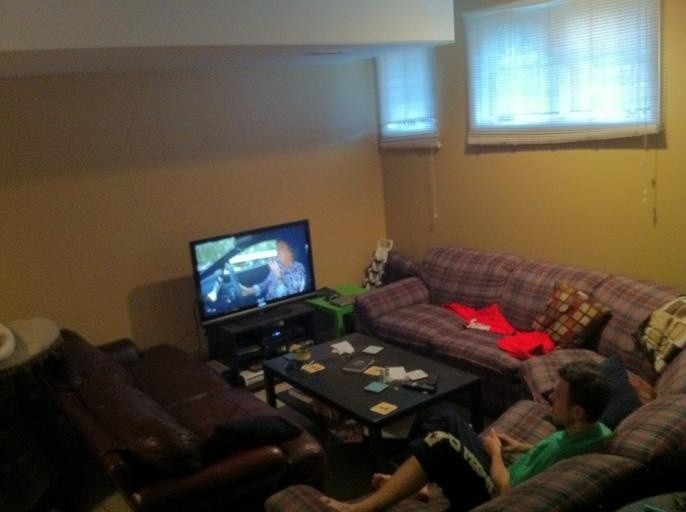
[267,246,685,512]
[17,329,326,512]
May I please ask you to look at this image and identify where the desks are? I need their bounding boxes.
[205,303,312,393]
[0,317,64,375]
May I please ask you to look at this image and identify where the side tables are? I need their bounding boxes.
[304,284,368,339]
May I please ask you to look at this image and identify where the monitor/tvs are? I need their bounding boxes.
[188,218,318,326]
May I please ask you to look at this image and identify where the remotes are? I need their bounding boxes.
[401,381,435,392]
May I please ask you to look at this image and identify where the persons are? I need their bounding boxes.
[316,358,616,512]
[233,234,309,303]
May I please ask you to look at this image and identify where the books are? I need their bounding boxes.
[342,355,374,375]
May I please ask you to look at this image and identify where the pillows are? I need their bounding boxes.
[529,278,610,349]
